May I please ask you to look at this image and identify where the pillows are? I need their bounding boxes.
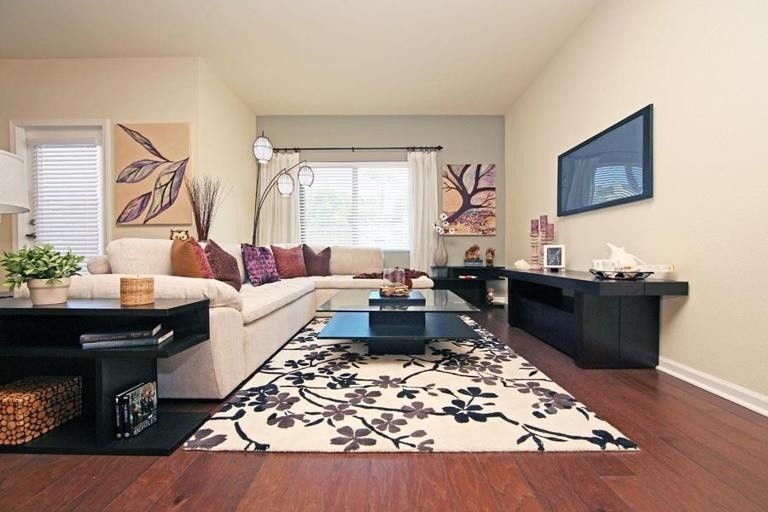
[168,240,331,295]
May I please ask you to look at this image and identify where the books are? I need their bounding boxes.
[80,322,161,344]
[115,381,146,438]
[124,380,159,437]
[82,329,174,350]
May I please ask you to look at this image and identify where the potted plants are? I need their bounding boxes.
[6,244,83,305]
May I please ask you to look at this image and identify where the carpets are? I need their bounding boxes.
[183,316,639,453]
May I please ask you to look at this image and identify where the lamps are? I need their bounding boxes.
[251,131,314,245]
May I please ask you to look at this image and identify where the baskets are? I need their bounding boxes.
[120,278,156,306]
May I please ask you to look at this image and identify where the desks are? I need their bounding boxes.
[5,296,210,455]
[433,264,504,307]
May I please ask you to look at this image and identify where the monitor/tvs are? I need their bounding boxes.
[556,104,654,217]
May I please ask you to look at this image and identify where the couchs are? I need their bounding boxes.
[65,235,433,400]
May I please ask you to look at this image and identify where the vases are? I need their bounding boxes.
[434,235,447,266]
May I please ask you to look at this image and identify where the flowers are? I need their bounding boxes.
[432,212,454,234]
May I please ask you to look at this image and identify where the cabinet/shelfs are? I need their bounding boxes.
[502,271,688,371]
